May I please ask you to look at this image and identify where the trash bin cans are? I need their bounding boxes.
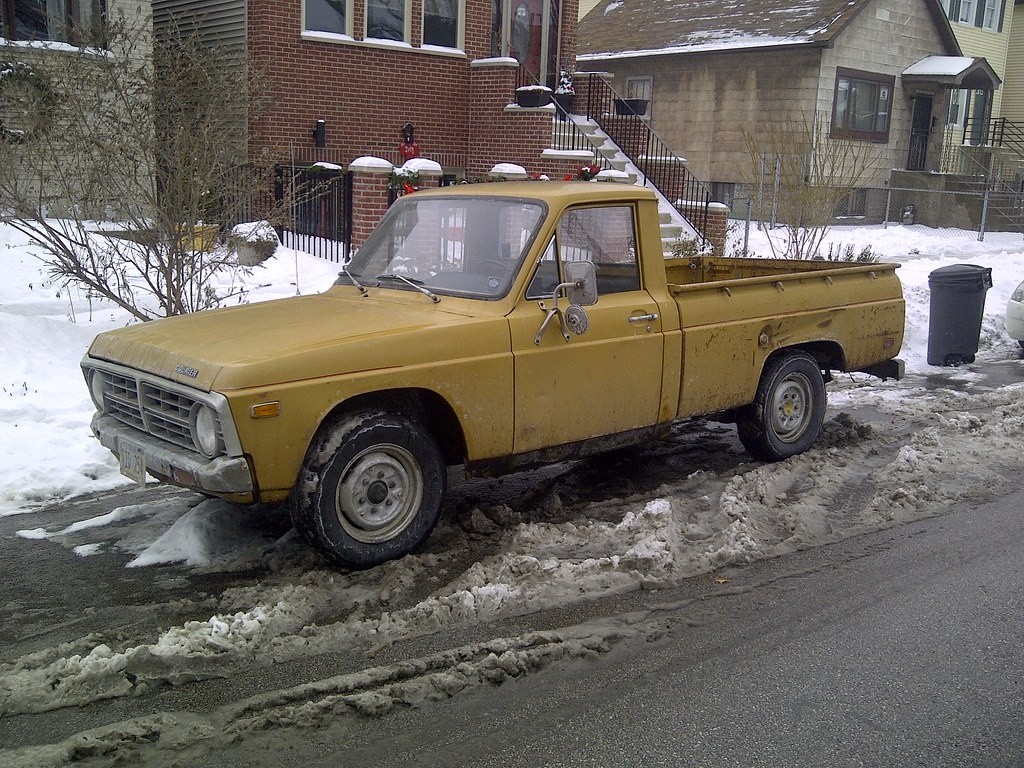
[927,262,994,368]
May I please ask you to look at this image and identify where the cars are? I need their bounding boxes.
[1007,280,1024,350]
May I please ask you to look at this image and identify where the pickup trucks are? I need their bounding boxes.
[78,180,908,571]
